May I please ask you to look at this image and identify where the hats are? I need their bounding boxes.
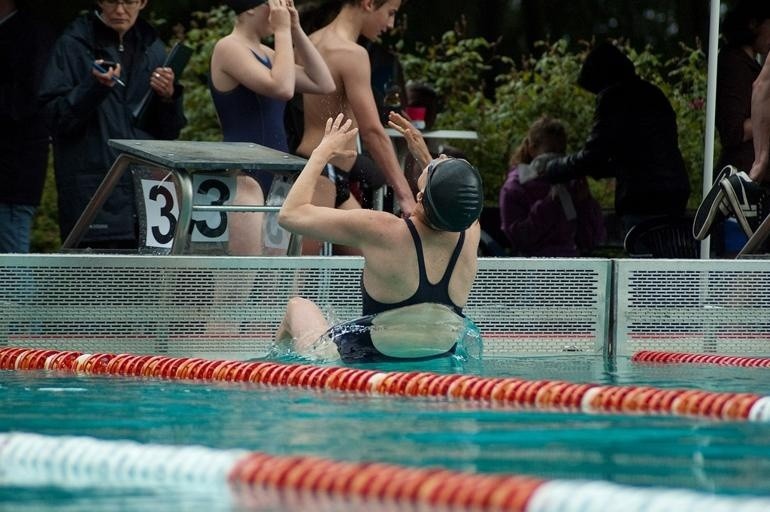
[421,157,484,232]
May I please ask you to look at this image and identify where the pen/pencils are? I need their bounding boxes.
[91,60,126,87]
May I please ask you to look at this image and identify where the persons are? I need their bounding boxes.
[205,0,336,333]
[499,43,687,260]
[716,1,770,260]
[277,0,483,362]
[0,0,49,335]
[32,0,184,334]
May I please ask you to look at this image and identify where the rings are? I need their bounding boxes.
[155,72,160,79]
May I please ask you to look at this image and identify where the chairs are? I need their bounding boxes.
[478,209,700,257]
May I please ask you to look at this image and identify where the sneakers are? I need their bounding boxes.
[690,164,741,241]
[719,169,768,240]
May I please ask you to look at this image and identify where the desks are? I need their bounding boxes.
[372,128,478,214]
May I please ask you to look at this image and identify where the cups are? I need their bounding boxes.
[403,106,428,130]
